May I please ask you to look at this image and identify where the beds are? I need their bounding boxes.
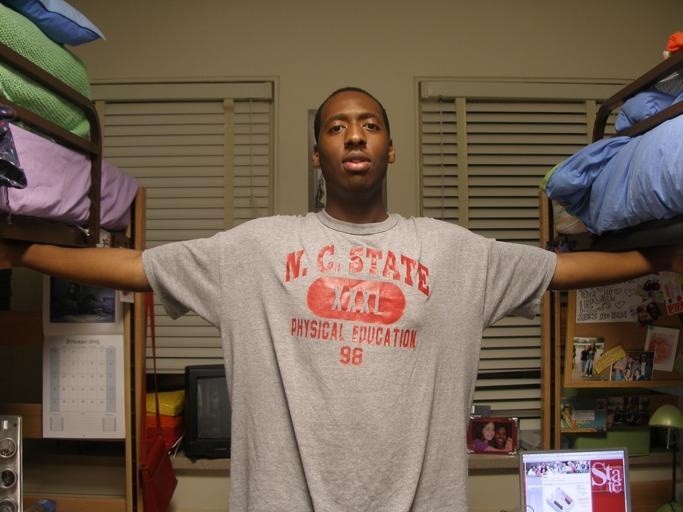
[0,43,146,512]
[538,46,683,512]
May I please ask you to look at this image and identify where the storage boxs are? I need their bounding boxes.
[569,425,651,457]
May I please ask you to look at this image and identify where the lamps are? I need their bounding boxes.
[648,404,683,512]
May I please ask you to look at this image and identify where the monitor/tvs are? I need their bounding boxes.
[519,447,631,512]
[184,364,231,459]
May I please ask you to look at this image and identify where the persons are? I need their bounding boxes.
[471,421,511,454]
[0,86,681,511]
[634,304,651,327]
[558,333,651,430]
[486,422,508,449]
[645,302,661,320]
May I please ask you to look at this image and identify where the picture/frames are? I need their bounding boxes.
[466,417,519,456]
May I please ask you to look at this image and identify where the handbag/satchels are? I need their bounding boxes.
[139,433,178,512]
